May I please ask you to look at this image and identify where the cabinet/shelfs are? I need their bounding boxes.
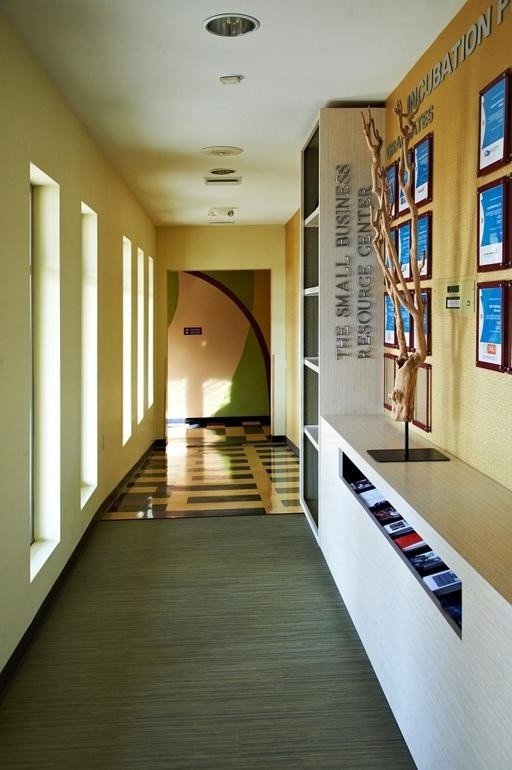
[300,108,384,545]
[317,417,512,768]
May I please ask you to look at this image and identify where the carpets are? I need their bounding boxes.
[2,513,416,762]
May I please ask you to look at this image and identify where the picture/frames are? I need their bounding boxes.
[475,67,511,176]
[475,280,507,373]
[383,130,432,357]
[383,353,398,411]
[477,176,509,273]
[411,362,431,433]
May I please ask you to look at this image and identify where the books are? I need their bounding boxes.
[348,478,463,596]
